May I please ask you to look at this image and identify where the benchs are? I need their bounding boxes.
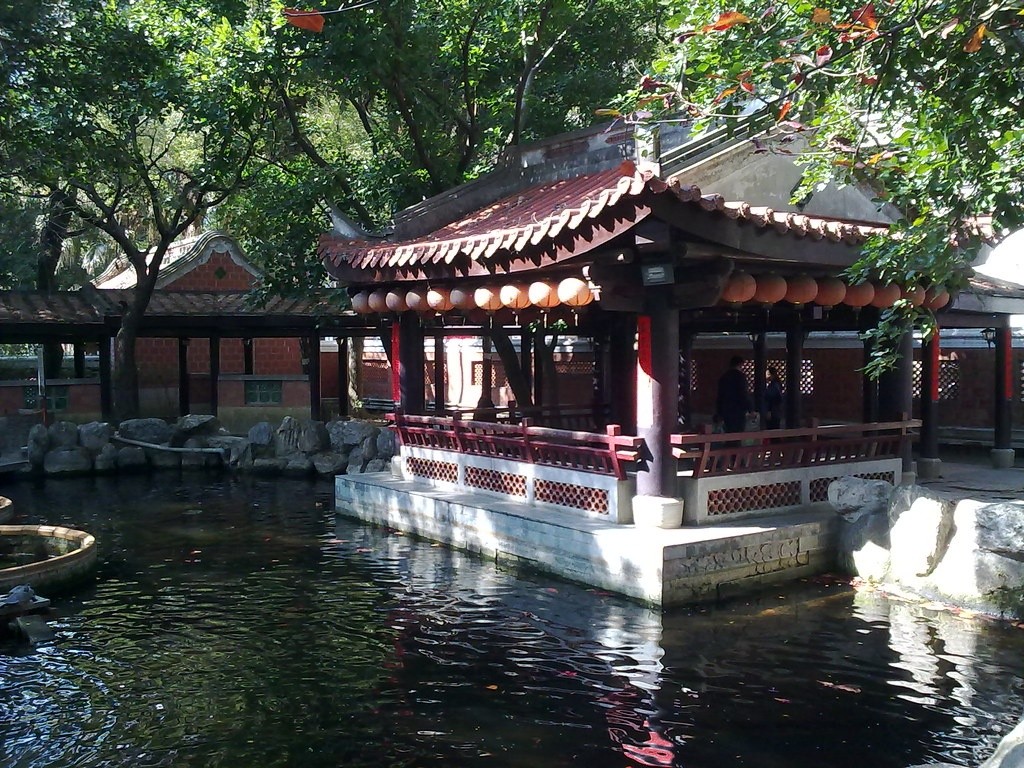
[396,404,923,472]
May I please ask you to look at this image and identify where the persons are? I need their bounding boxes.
[712,355,757,451]
[765,368,783,429]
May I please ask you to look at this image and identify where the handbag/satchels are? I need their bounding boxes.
[740,411,762,447]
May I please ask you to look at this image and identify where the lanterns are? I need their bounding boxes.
[722,273,756,305]
[753,274,787,309]
[405,288,431,328]
[904,285,925,308]
[870,281,902,308]
[448,284,476,326]
[385,290,410,324]
[922,286,950,311]
[352,292,370,315]
[368,290,390,324]
[472,281,504,330]
[785,274,819,305]
[427,287,455,327]
[527,277,561,329]
[841,281,875,312]
[557,273,595,328]
[499,280,532,327]
[815,277,845,310]
